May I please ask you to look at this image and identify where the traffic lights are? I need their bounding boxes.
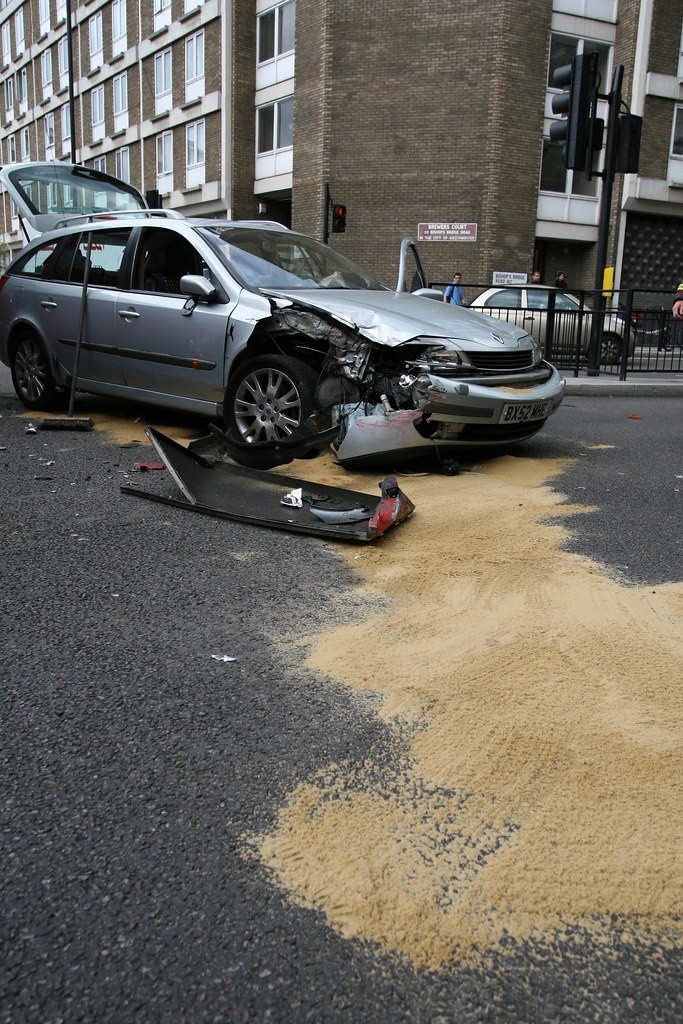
[547,53,593,174]
[332,205,346,233]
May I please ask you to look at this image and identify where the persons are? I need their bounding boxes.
[528,272,541,284]
[443,273,464,306]
[671,281,683,320]
[555,273,568,288]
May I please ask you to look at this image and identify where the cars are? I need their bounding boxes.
[0,159,567,471]
[465,282,638,367]
